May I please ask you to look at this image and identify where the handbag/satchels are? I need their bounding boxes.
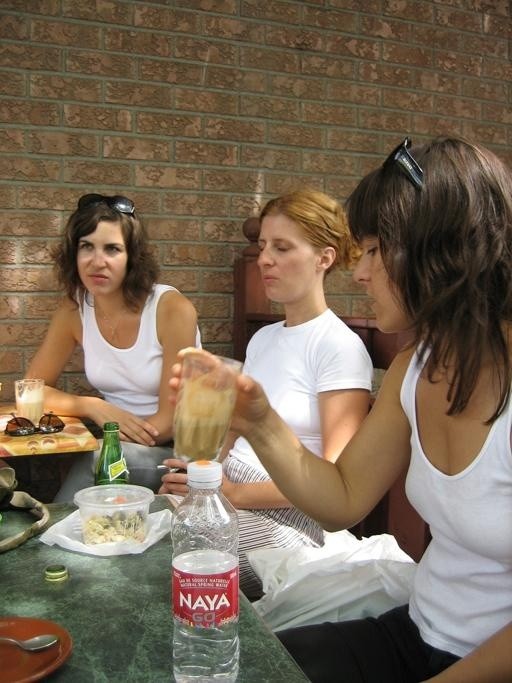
[0,459,16,509]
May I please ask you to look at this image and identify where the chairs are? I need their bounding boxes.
[350,398,426,563]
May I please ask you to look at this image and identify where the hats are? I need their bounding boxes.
[78,192,138,219]
[384,137,424,191]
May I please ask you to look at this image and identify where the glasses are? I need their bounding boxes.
[5,411,68,435]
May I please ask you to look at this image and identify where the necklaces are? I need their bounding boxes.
[99,306,124,342]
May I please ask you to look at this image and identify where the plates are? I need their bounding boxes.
[0,618,72,682]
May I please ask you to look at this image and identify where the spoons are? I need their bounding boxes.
[1,634,59,651]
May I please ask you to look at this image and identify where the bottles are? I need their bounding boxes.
[94,422,129,486]
[171,457,241,681]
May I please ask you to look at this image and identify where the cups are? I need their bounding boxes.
[14,379,45,428]
[173,351,244,462]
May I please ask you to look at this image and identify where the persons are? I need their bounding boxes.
[169,133,512,682]
[23,193,204,504]
[156,186,377,604]
